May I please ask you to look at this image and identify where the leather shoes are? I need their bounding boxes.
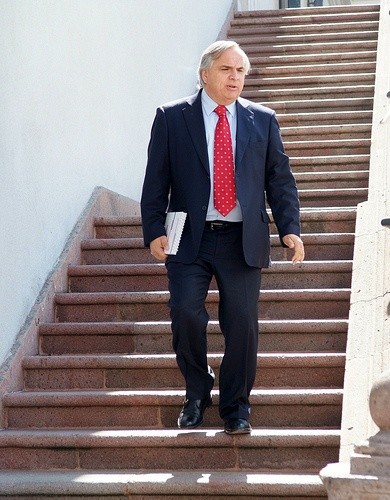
[224,419,252,435]
[178,396,213,430]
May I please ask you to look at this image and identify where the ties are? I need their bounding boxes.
[213,105,238,217]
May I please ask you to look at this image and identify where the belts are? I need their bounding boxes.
[205,221,243,232]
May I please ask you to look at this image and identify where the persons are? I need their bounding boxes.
[140,40,305,434]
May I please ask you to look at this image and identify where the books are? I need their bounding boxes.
[164,211,188,256]
